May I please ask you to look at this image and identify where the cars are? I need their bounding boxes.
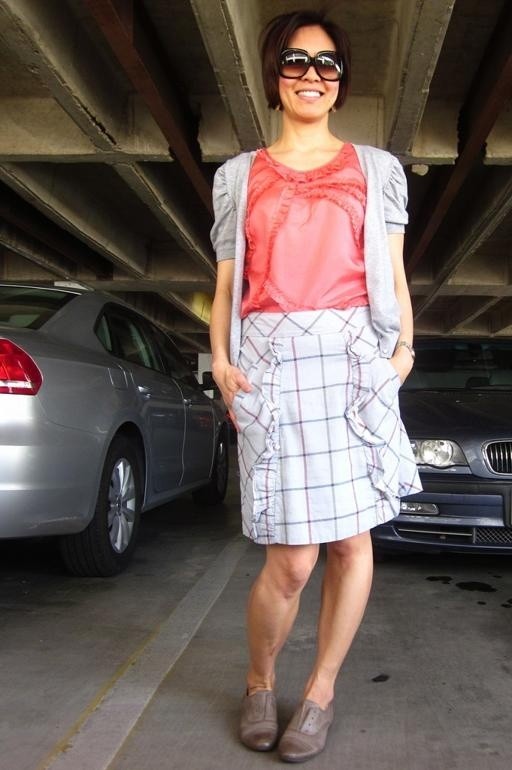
[369,329,512,568]
[1,270,236,581]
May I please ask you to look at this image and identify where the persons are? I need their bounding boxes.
[207,9,419,764]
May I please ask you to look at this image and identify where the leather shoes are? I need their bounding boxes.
[240,685,279,750]
[278,698,333,763]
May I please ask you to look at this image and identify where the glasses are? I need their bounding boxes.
[273,47,344,82]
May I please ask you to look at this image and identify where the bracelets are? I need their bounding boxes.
[395,341,416,358]
